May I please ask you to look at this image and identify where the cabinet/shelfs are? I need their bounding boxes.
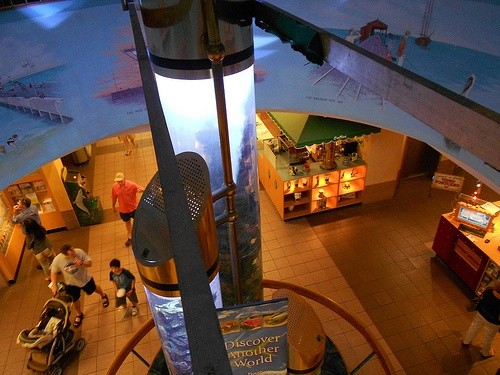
[431,200,500,299]
[256,111,368,225]
[0,157,81,292]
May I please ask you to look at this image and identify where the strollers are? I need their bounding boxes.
[16,292,86,375]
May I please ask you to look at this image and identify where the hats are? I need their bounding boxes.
[114,172,125,181]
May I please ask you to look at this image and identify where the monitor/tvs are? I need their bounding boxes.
[454,202,494,238]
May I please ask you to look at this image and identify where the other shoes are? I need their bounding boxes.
[37,265,43,270]
[125,238,132,246]
[44,276,51,281]
[121,311,128,320]
[132,306,139,316]
[461,340,471,350]
[481,349,495,358]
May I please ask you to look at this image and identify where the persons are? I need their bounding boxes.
[108,258,140,317]
[48,243,109,328]
[119,132,138,156]
[10,197,56,281]
[111,171,146,247]
[460,270,500,357]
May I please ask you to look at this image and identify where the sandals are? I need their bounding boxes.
[73,312,84,328]
[101,293,109,308]
